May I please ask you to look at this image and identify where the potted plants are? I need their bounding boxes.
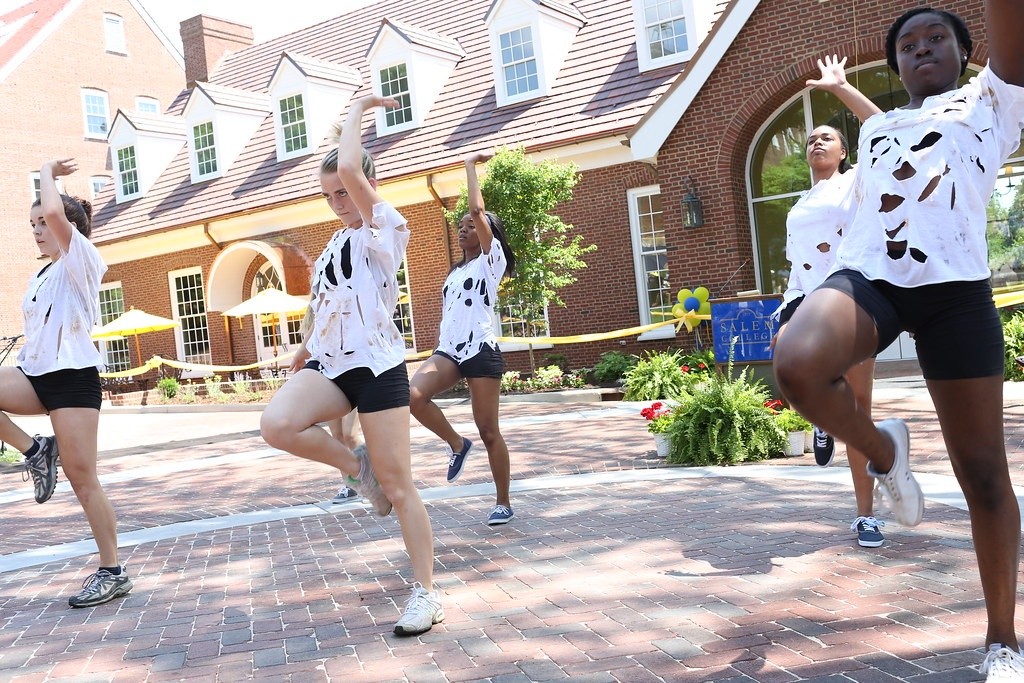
[574,349,632,388]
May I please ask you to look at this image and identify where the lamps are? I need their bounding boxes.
[256,253,263,287]
[681,177,702,229]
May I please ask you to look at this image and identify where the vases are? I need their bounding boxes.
[780,430,806,456]
[704,377,712,391]
[694,381,702,392]
[806,429,817,453]
[654,430,673,457]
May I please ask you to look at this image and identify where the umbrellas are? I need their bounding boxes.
[220,282,310,373]
[93,305,180,379]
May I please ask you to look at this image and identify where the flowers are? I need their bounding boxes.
[619,345,815,436]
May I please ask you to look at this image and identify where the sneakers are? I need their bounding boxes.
[445,436,474,483]
[392,581,446,636]
[332,486,359,504]
[850,515,885,548]
[979,643,1024,683]
[486,505,515,525]
[68,565,134,607]
[866,419,924,527]
[812,426,835,468]
[346,441,392,516]
[21,433,59,504]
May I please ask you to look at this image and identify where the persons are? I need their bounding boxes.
[0,156,134,607]
[259,95,444,636]
[409,152,519,526]
[766,0,1024,683]
[768,54,886,550]
